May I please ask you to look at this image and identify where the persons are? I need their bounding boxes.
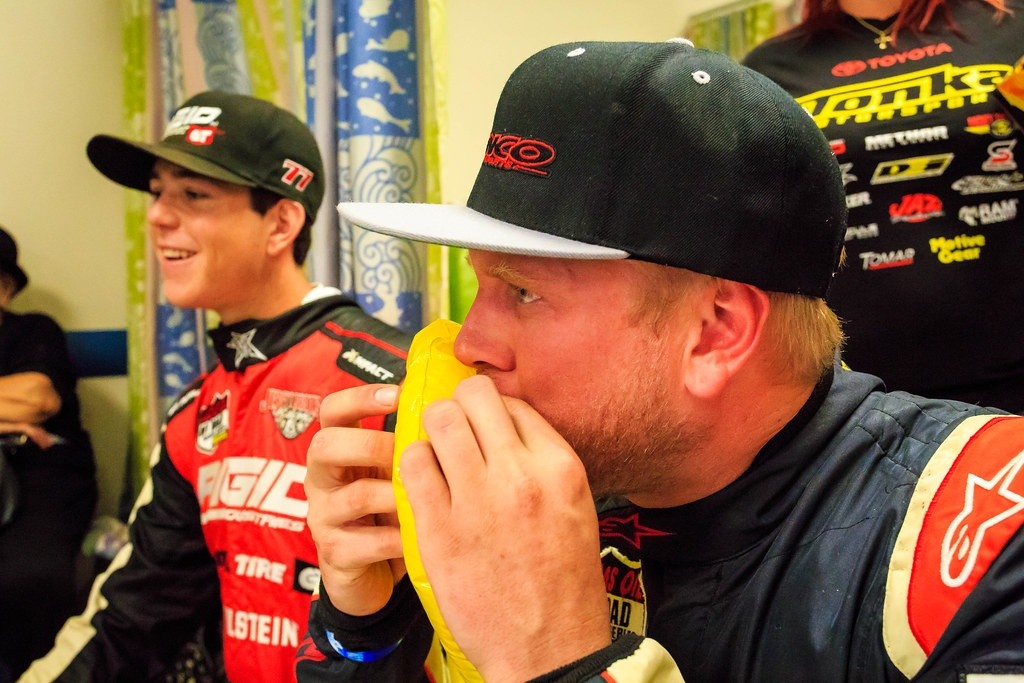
[294,36,1024,683]
[739,0,1024,416]
[0,90,413,680]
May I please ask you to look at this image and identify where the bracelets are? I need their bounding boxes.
[323,629,407,662]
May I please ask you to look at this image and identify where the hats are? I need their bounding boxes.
[332,40,848,302]
[0,225,31,298]
[86,91,325,224]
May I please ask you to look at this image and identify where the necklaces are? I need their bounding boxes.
[855,15,893,49]
